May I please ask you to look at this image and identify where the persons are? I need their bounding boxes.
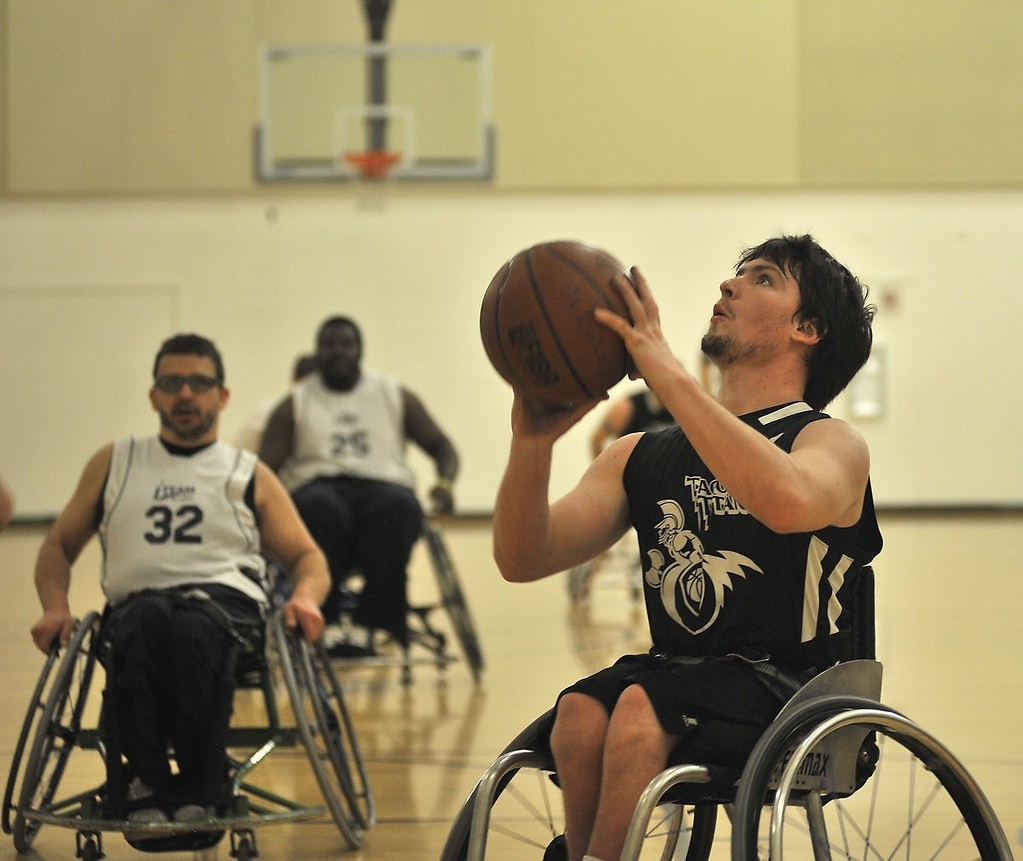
[258,318,459,659]
[492,235,883,861]
[31,335,329,851]
[591,388,676,456]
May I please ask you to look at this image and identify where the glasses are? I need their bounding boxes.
[155,374,218,393]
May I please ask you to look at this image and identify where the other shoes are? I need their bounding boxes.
[125,803,224,852]
[323,624,369,657]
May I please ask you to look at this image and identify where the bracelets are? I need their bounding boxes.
[436,478,454,488]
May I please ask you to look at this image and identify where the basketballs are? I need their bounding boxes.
[479,241,636,409]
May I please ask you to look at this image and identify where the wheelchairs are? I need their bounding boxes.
[560,544,646,607]
[272,513,486,678]
[0,611,373,861]
[434,565,1012,861]
